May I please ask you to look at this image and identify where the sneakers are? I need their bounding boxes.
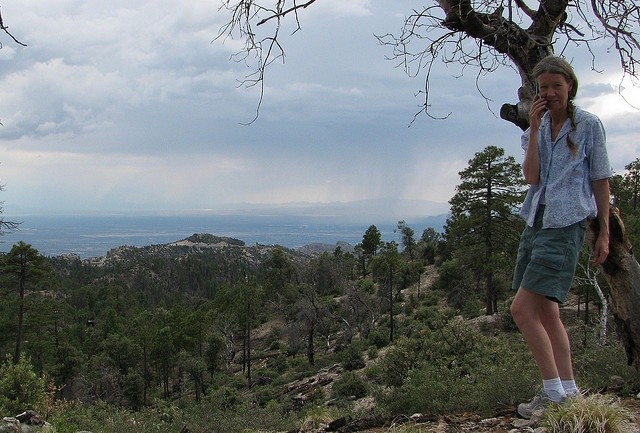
[517,394,569,419]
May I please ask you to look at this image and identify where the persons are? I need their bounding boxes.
[509,55,612,418]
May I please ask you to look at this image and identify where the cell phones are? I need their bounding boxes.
[535,85,540,101]
